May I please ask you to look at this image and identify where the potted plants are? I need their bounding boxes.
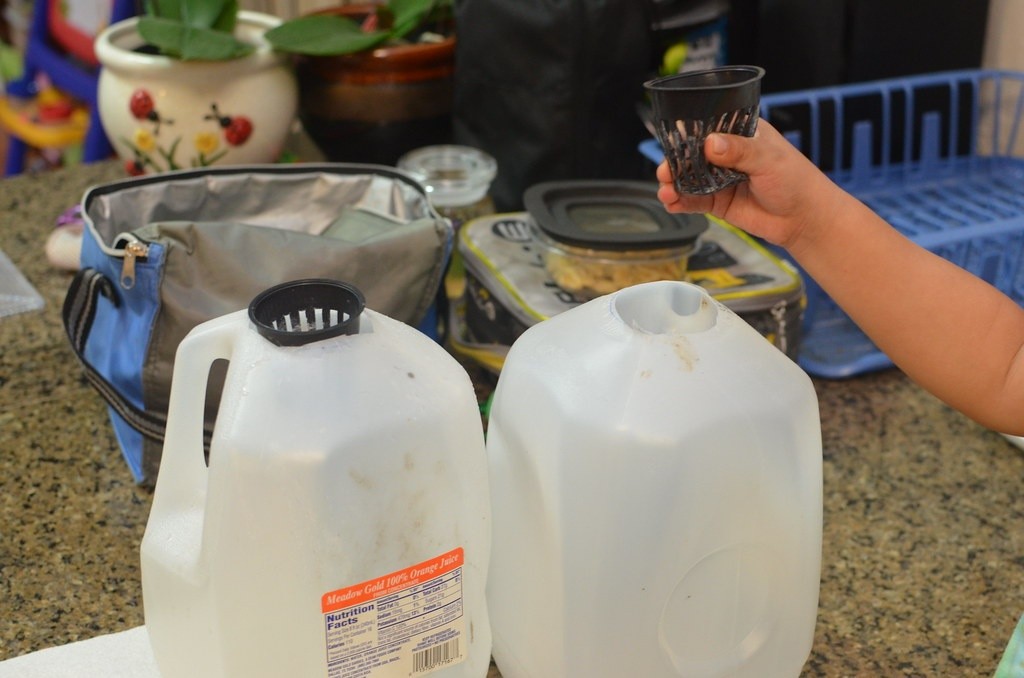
[263,1,458,165]
[91,1,298,175]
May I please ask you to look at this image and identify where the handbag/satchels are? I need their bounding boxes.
[443,211,807,393]
[60,162,454,490]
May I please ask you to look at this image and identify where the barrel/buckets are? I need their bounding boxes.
[487,281,825,678]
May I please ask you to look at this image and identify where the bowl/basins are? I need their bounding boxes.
[513,181,709,303]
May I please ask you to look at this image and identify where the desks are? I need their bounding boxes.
[0,122,1023,678]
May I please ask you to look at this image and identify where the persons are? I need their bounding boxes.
[657,115,1024,440]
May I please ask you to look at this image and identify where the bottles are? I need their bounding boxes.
[397,146,500,336]
[139,279,491,678]
